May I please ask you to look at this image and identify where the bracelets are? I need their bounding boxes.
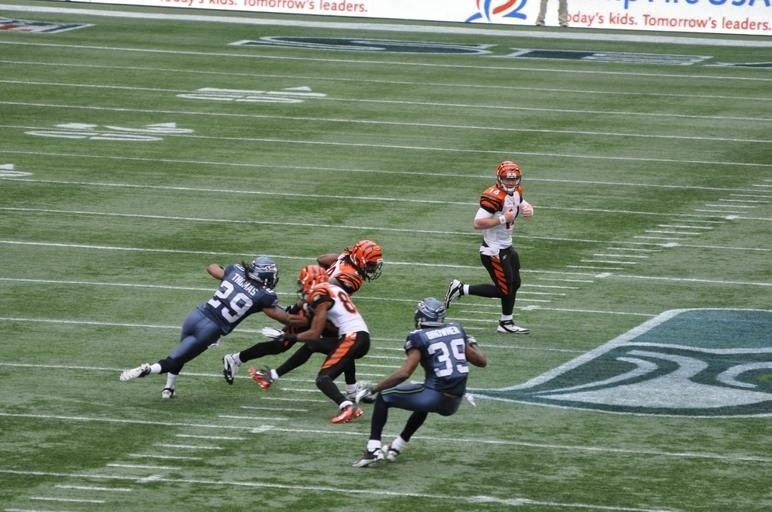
[284,334,297,341]
[499,215,506,224]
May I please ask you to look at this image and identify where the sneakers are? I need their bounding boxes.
[331,383,375,423]
[120,364,151,381]
[223,354,275,389]
[444,279,463,308]
[497,320,530,333]
[161,388,176,399]
[352,445,399,468]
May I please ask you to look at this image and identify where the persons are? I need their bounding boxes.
[352,296,487,468]
[247,264,370,424]
[536,0,568,28]
[119,256,289,400]
[222,240,384,404]
[445,160,534,334]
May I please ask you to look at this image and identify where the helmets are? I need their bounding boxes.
[297,265,328,296]
[249,257,277,284]
[414,297,445,328]
[496,161,521,193]
[352,240,383,279]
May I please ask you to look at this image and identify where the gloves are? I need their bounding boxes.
[262,327,286,341]
[355,389,372,404]
